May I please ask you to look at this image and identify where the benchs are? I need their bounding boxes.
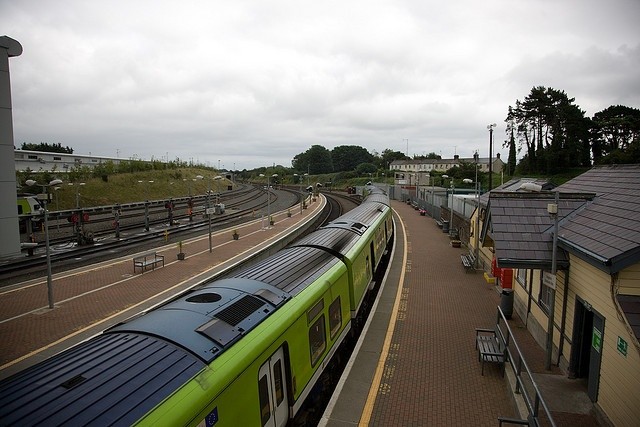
[448,227,459,239]
[477,330,505,379]
[411,201,417,208]
[461,250,477,274]
[436,216,445,227]
[498,414,537,426]
[133,251,164,274]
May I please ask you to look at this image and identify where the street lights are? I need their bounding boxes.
[442,175,454,230]
[292,173,309,215]
[259,173,279,229]
[426,174,436,219]
[463,178,481,269]
[138,180,154,201]
[487,122,498,190]
[183,178,197,196]
[195,174,222,253]
[24,179,64,308]
[67,181,86,209]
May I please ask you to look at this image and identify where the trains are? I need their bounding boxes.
[0,184,393,426]
[17,191,48,233]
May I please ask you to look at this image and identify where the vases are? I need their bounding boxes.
[420,212,425,216]
[452,241,461,247]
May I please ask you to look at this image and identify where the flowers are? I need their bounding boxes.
[419,209,426,213]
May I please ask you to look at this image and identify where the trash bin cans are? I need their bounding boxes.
[443,220,449,233]
[501,288,515,320]
